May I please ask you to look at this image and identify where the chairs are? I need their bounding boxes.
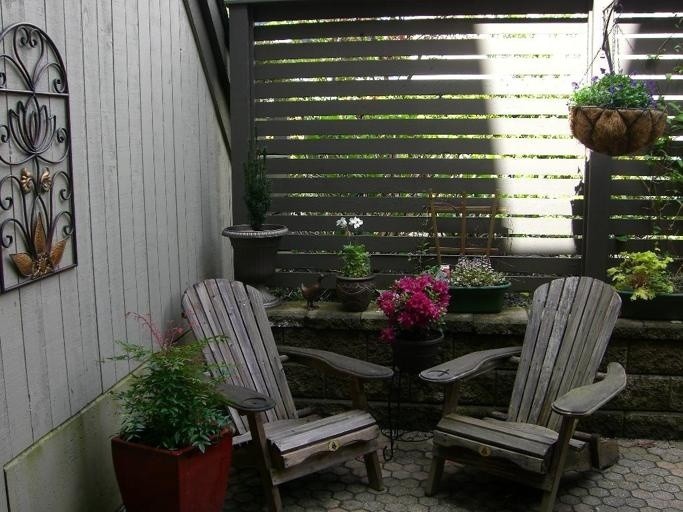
[417,275,628,512]
[183,276,397,511]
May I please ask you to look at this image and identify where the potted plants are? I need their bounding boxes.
[218,142,288,285]
[103,306,241,512]
[608,251,682,319]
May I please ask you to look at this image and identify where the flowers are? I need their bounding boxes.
[330,215,372,274]
[439,253,507,286]
[569,67,662,113]
[375,269,452,340]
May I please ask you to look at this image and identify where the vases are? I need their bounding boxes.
[334,274,373,311]
[570,106,666,158]
[401,328,444,374]
[446,283,511,315]
